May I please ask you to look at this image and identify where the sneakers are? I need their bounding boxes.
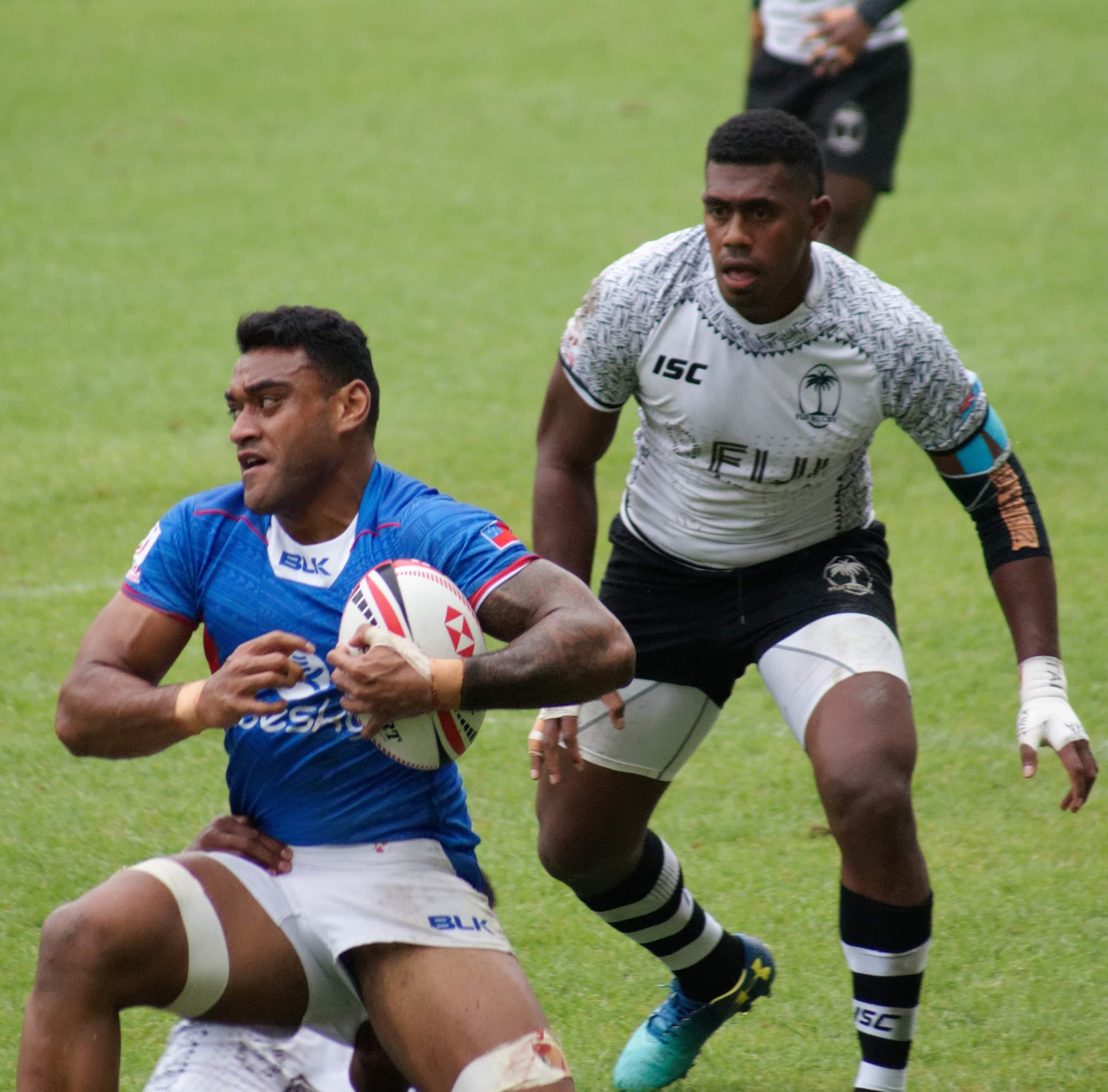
[613,934,775,1092]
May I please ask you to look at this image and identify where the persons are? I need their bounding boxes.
[534,101,1097,1090]
[18,307,637,1091]
[746,1,912,261]
[146,815,354,1090]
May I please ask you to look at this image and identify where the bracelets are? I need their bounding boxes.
[432,656,463,710]
[176,678,204,734]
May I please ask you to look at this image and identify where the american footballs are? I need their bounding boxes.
[338,557,487,771]
